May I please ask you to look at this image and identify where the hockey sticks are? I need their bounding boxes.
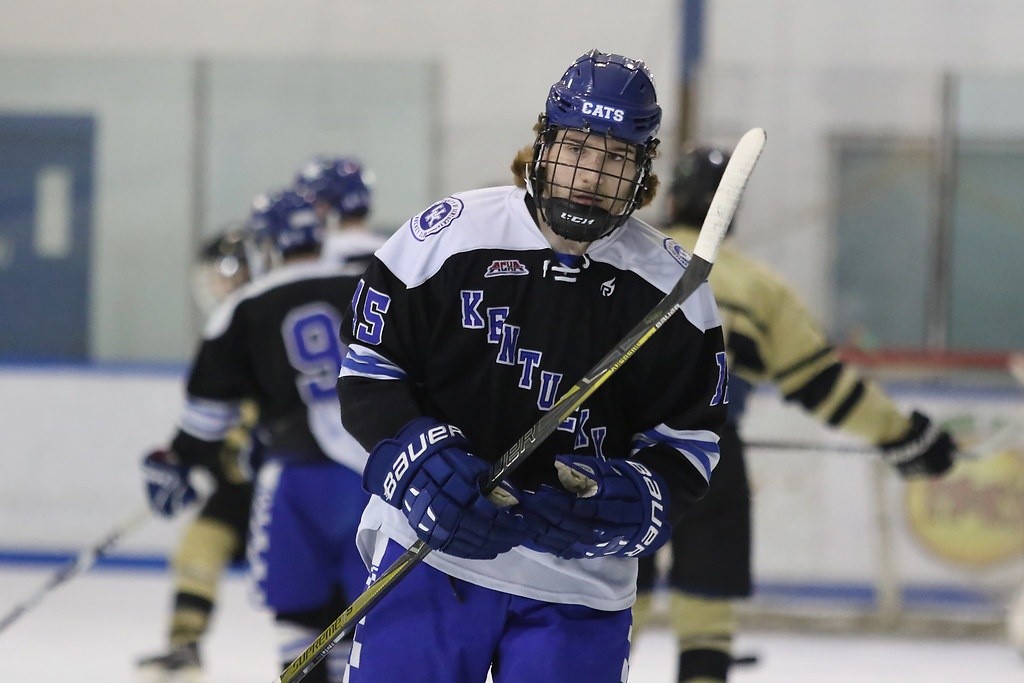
[1,499,164,634]
[276,123,767,683]
[741,417,1024,465]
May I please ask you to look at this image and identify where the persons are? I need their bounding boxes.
[136,156,388,683]
[631,145,962,683]
[339,48,730,683]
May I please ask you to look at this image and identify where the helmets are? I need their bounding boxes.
[526,47,662,242]
[670,144,737,232]
[247,188,321,250]
[293,156,372,220]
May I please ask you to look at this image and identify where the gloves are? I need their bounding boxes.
[875,410,960,480]
[361,419,528,557]
[144,451,198,514]
[518,454,672,561]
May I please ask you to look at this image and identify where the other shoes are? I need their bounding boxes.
[139,640,202,678]
